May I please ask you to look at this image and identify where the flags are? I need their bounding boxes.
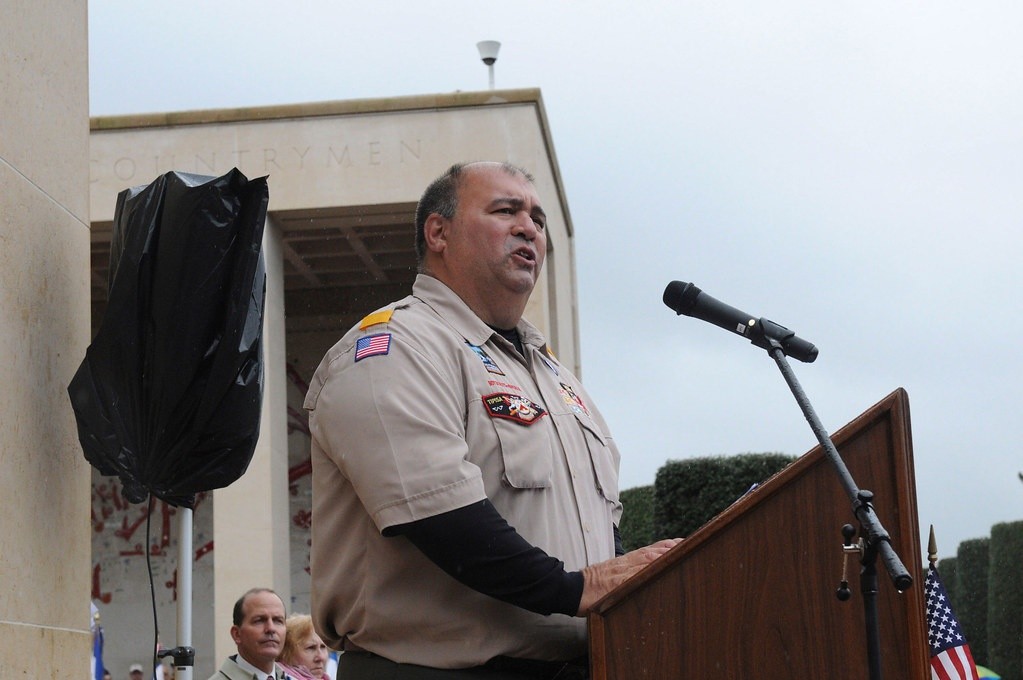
[923,564,979,680]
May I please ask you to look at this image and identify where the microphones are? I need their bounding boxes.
[663,280,819,363]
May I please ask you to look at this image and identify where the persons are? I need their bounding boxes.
[129,663,144,680]
[207,588,334,680]
[302,160,686,680]
